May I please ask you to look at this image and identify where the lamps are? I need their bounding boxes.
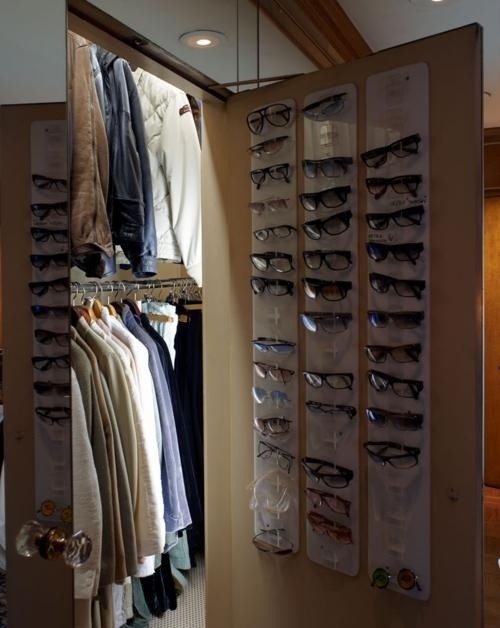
[179,30,228,50]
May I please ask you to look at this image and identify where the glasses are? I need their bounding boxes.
[32,296,75,319]
[35,404,73,427]
[298,452,355,490]
[251,382,294,411]
[30,353,75,373]
[298,208,355,241]
[30,171,71,195]
[247,134,291,157]
[32,377,72,399]
[363,305,426,332]
[252,357,296,387]
[250,274,295,297]
[33,498,74,524]
[300,247,354,271]
[243,101,293,136]
[295,182,352,213]
[364,238,426,266]
[247,161,292,189]
[362,438,423,468]
[305,508,355,546]
[252,415,295,439]
[254,222,293,242]
[251,525,297,558]
[298,154,355,180]
[29,198,74,221]
[250,249,295,274]
[363,341,425,365]
[299,367,356,394]
[255,440,297,477]
[359,131,424,170]
[364,404,427,433]
[304,395,359,422]
[29,275,72,297]
[247,196,291,216]
[364,562,426,597]
[29,224,72,245]
[26,247,71,273]
[367,270,427,303]
[303,484,351,518]
[298,306,357,334]
[365,368,424,402]
[301,273,355,302]
[33,324,75,350]
[298,91,353,121]
[363,202,426,232]
[251,334,296,355]
[363,173,427,200]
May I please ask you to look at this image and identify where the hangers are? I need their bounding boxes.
[70,278,203,323]
